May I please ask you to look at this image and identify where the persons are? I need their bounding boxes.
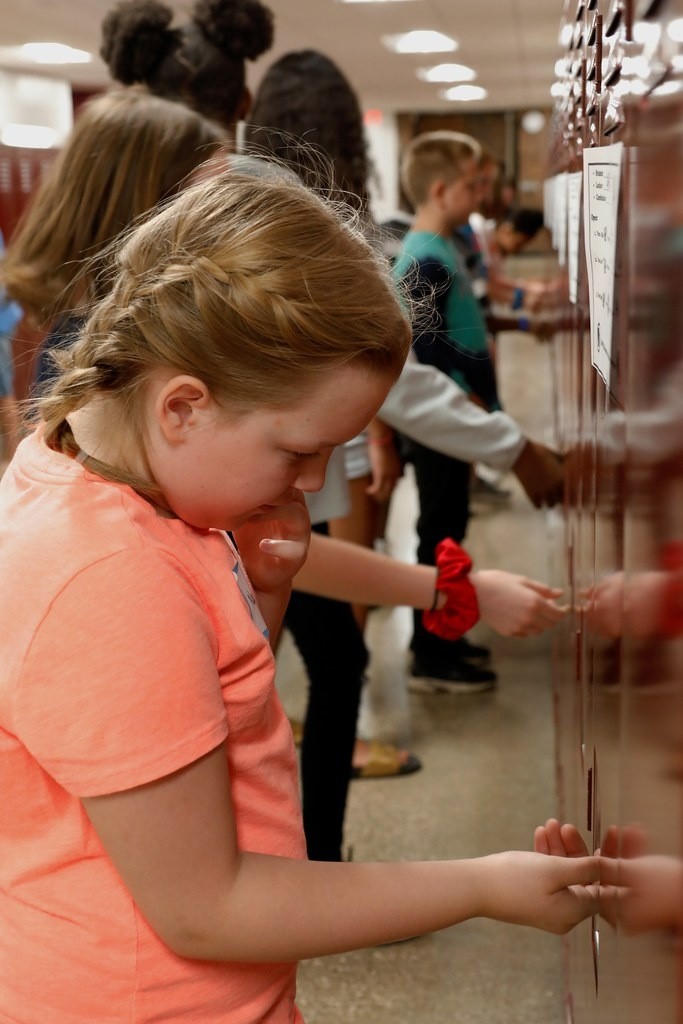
[0,152,603,1024]
[385,131,567,699]
[100,1,278,133]
[243,45,573,943]
[1,83,234,474]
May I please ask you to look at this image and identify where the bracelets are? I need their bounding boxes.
[421,536,480,641]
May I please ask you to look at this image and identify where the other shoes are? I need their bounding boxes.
[450,637,490,665]
[353,737,422,778]
[409,654,497,693]
